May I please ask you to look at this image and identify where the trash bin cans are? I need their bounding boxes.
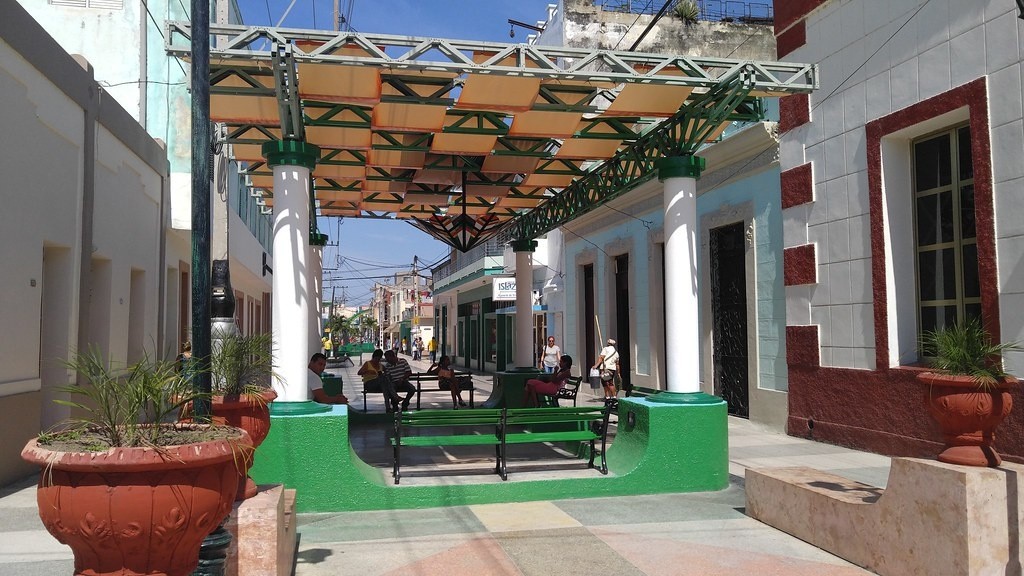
[588,376,601,389]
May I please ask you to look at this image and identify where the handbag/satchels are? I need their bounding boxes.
[590,366,599,377]
[412,345,417,351]
[421,347,424,351]
[541,361,546,372]
[601,370,613,381]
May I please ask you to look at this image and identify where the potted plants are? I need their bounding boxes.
[20,340,253,576]
[164,323,285,499]
[915,312,1024,466]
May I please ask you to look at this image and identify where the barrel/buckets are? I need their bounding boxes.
[588,367,601,389]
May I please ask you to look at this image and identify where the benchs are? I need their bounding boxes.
[361,371,474,411]
[389,399,618,485]
[525,374,583,407]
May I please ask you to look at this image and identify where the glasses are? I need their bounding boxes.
[549,340,553,342]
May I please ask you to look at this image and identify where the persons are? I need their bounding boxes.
[592,339,620,402]
[402,336,408,354]
[183,342,194,383]
[540,336,560,373]
[521,355,572,408]
[394,336,399,350]
[386,336,390,349]
[412,337,423,360]
[358,349,404,414]
[384,350,415,412]
[375,335,379,346]
[322,336,332,357]
[427,356,467,410]
[427,337,438,364]
[307,353,350,403]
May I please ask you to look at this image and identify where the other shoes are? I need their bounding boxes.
[386,408,398,417]
[613,398,618,402]
[454,406,458,410]
[602,397,610,402]
[459,401,466,406]
[402,402,407,411]
[394,395,402,401]
